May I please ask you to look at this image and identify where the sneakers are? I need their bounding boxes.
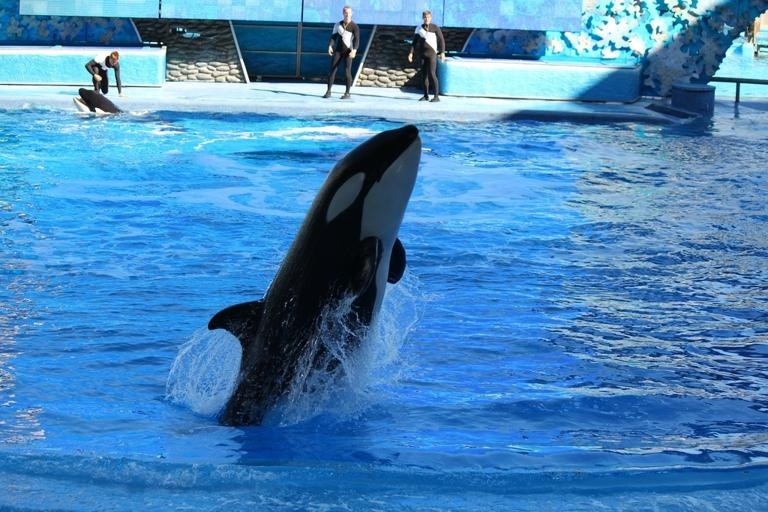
[324,92,331,98]
[342,93,350,99]
[419,96,439,102]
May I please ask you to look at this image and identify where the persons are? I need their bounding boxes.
[407,9,446,103]
[84,50,122,98]
[321,6,360,99]
